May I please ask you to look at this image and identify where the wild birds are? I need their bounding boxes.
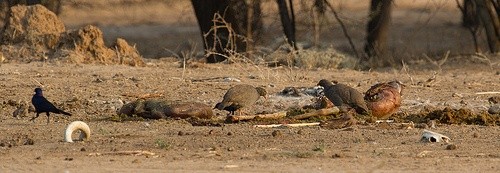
[317,79,369,117]
[364,79,409,120]
[212,85,268,117]
[28,87,72,124]
